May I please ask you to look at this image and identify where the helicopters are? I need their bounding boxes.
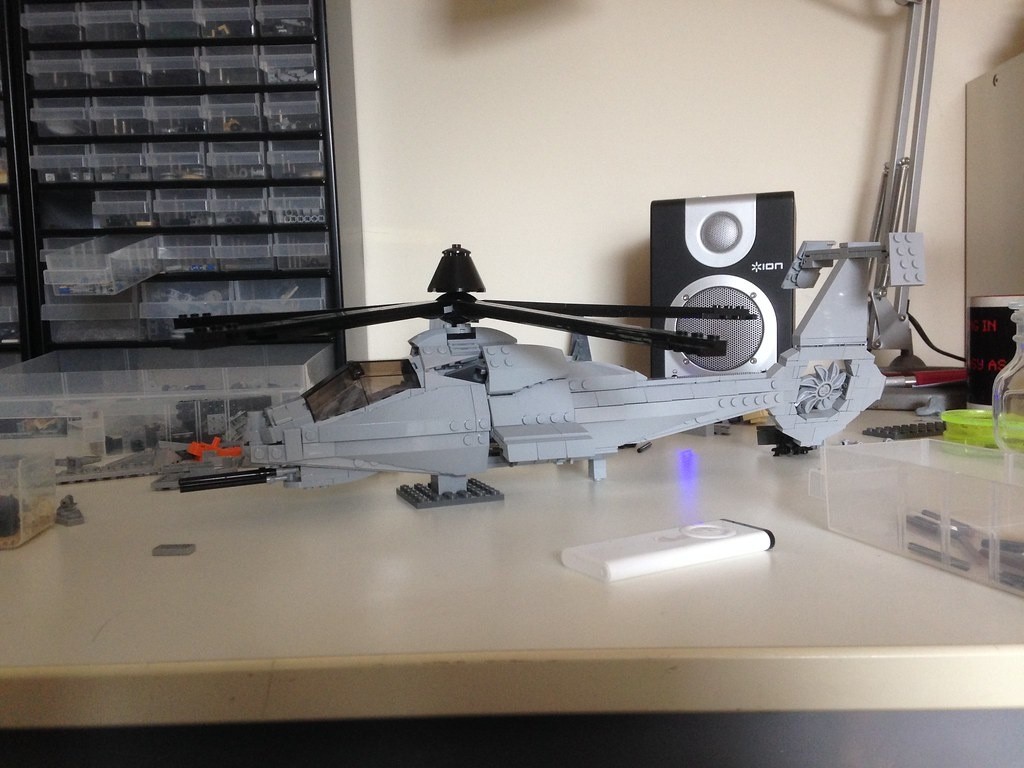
[166,232,924,498]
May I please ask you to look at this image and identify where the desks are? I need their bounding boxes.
[0,410,1024,728]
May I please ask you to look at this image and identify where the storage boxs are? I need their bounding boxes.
[20,0,330,340]
[0,450,55,549]
[0,343,335,472]
[819,439,1024,598]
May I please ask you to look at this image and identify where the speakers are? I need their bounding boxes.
[648,191,803,387]
